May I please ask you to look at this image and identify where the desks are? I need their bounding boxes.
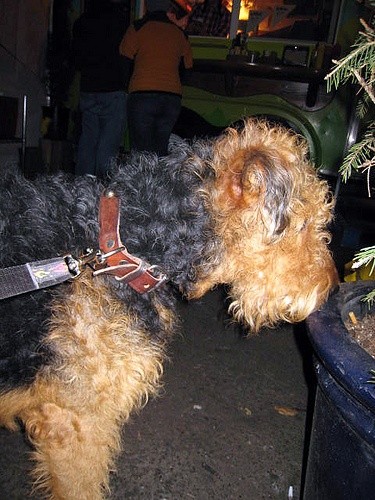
[176,63,362,227]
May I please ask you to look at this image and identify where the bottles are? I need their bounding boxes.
[320,39,334,74]
[329,40,341,73]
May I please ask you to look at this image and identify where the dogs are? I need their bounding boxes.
[0,120,338,499]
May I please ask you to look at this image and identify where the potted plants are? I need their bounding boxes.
[283,18,375,500]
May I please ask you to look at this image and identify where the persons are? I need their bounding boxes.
[119,0,194,163]
[71,0,131,181]
